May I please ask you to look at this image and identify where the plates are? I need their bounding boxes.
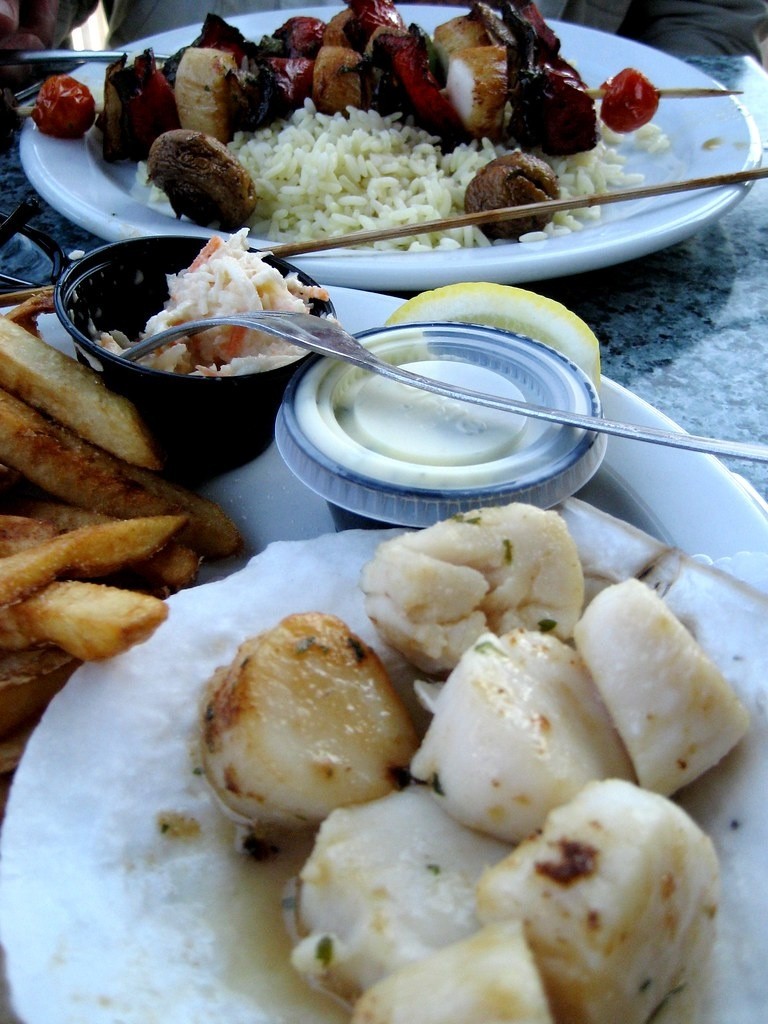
[0,286,768,570]
[19,2,763,290]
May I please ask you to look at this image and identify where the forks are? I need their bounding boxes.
[117,310,768,463]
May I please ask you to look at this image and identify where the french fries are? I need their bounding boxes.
[2,312,236,807]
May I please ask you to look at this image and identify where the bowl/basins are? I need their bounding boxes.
[276,321,609,531]
[54,235,335,464]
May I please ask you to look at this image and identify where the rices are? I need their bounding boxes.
[137,98,667,247]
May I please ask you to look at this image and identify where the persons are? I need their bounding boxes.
[0,1,353,79]
